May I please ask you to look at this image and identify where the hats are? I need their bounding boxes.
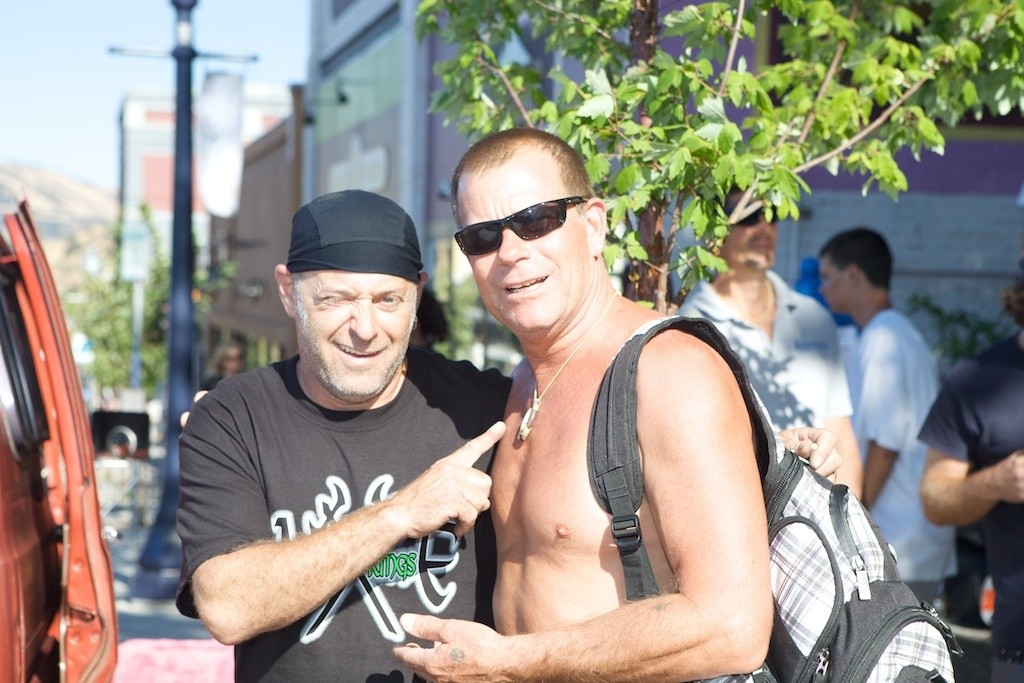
[287,190,423,283]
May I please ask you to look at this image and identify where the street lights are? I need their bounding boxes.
[106,42,263,597]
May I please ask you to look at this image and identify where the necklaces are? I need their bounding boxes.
[515,291,621,442]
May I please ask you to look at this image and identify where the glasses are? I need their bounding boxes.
[739,205,780,226]
[454,195,590,256]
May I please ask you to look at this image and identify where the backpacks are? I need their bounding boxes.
[585,317,963,683]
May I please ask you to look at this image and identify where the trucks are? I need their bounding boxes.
[0,180,116,683]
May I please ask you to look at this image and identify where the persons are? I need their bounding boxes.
[199,342,249,405]
[677,175,862,513]
[175,190,842,683]
[181,128,776,683]
[820,227,957,622]
[917,247,1024,683]
